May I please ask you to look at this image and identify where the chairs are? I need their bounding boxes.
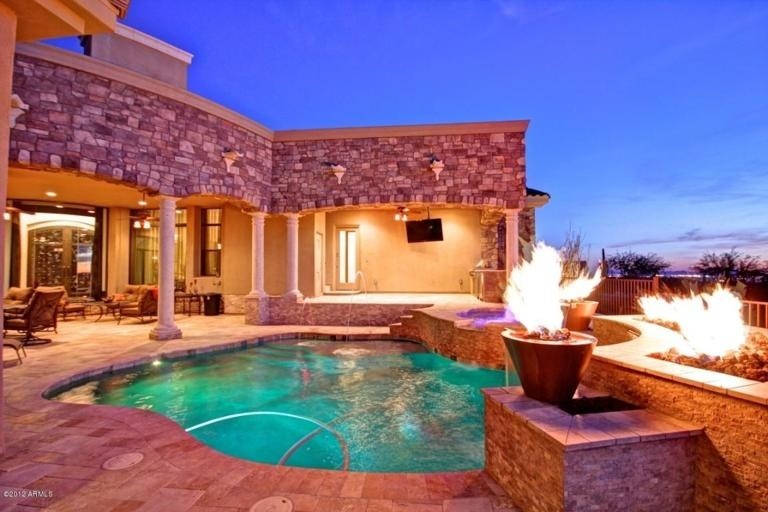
[0,282,158,365]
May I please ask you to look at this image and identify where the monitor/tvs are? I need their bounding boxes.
[405,218,443,243]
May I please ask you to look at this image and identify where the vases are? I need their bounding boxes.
[561,300,598,330]
[501,327,598,404]
